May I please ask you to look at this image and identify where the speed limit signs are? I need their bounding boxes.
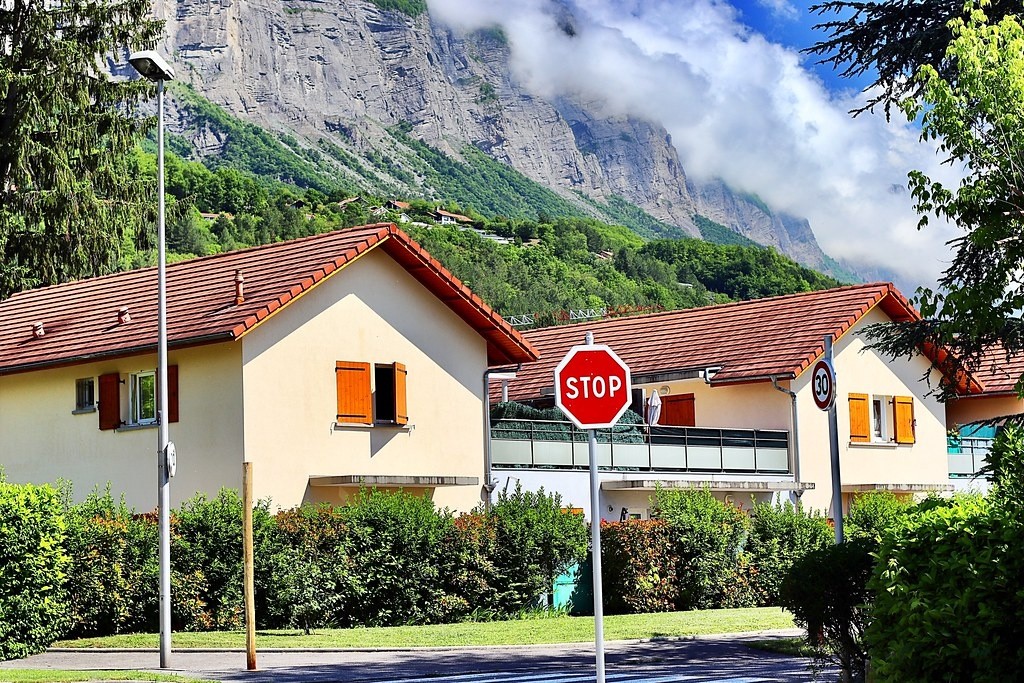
[811,360,833,410]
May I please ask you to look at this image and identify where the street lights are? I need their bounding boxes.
[127,50,176,668]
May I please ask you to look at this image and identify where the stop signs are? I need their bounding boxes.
[554,345,633,429]
